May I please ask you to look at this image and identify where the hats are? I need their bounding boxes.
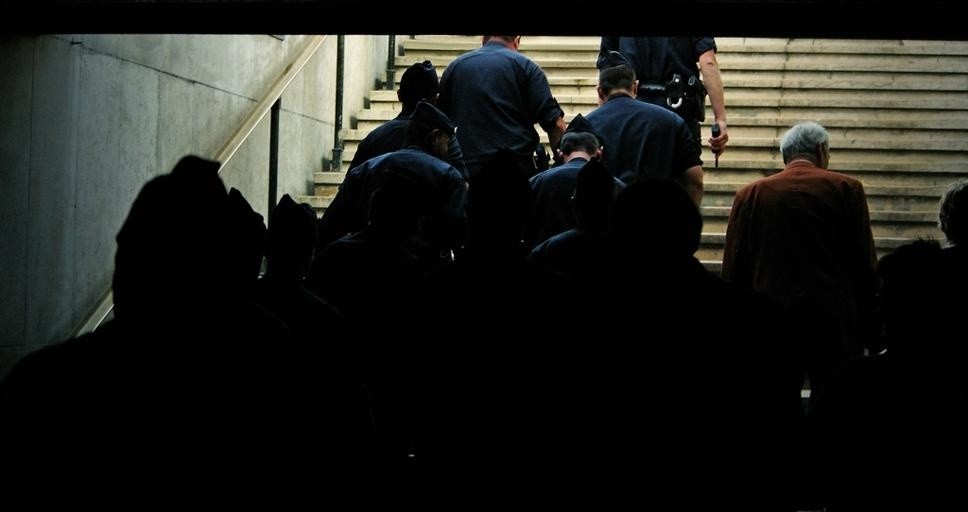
[557,114,604,151]
[406,98,455,138]
[598,49,629,69]
[400,61,439,97]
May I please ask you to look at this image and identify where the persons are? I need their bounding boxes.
[2,98,968,512]
[587,48,702,205]
[437,35,566,179]
[348,61,471,180]
[601,33,706,136]
